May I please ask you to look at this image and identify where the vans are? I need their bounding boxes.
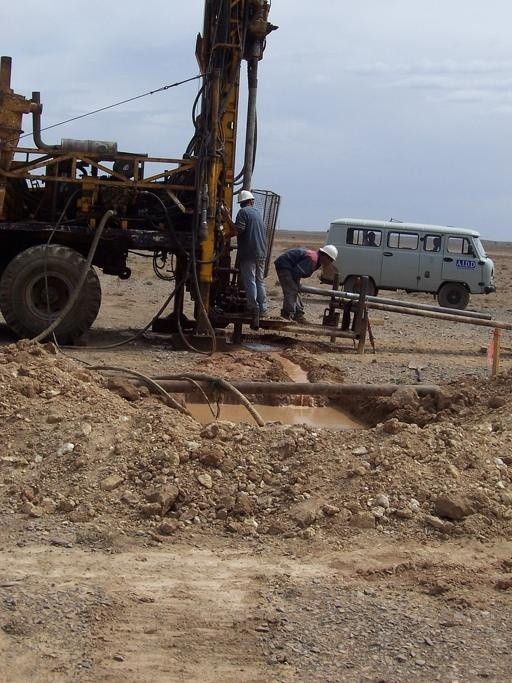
[320,221,494,309]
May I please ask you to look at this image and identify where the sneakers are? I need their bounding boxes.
[281,311,313,323]
[244,309,268,317]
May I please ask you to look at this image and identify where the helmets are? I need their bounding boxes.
[320,245,338,261]
[239,190,254,203]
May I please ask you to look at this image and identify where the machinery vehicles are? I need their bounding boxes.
[1,2,278,345]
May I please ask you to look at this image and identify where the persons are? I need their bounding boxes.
[233,190,268,317]
[274,244,338,325]
[367,231,378,247]
